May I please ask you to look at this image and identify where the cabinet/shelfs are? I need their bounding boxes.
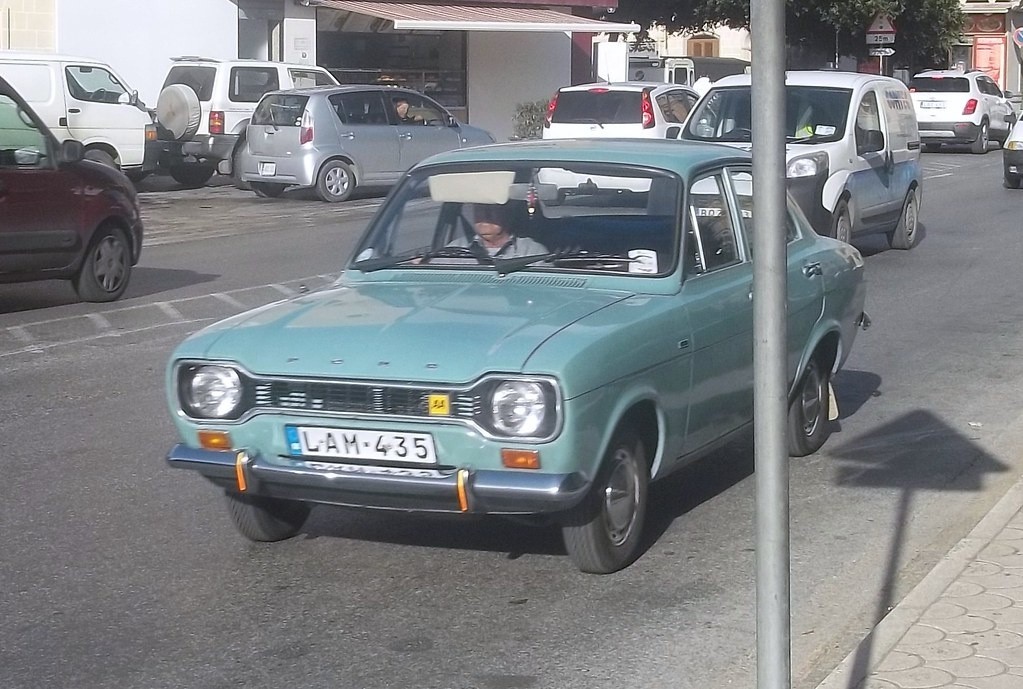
[328,69,465,106]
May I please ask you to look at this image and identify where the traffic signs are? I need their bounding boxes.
[869,49,895,56]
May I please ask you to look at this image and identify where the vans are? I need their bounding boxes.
[0,51,158,199]
[666,71,923,250]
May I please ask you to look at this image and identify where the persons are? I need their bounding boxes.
[395,98,423,122]
[413,199,554,267]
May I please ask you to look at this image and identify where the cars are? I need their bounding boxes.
[164,140,869,576]
[0,75,142,303]
[1002,110,1023,188]
[233,85,495,202]
[536,81,718,219]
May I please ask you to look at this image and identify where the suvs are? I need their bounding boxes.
[901,68,1018,154]
[154,56,345,186]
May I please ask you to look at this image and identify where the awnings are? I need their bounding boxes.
[313,0,641,33]
